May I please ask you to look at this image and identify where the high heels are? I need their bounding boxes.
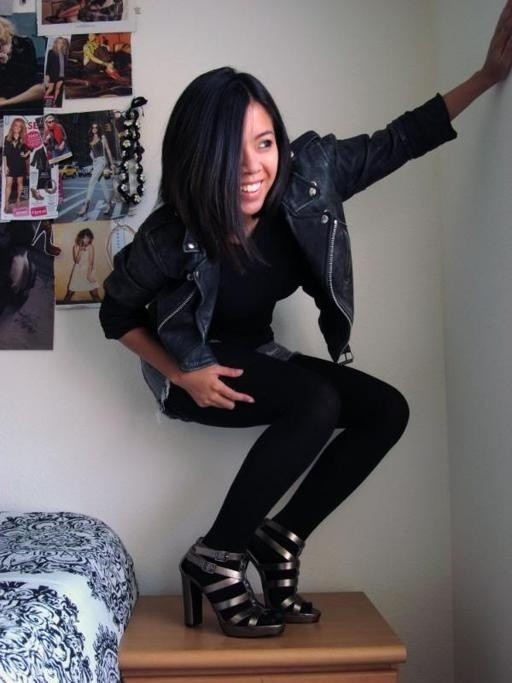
[246,518,322,623]
[179,537,286,639]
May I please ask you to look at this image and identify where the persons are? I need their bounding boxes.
[83,33,118,73]
[99,0,512,639]
[43,115,69,158]
[0,17,44,107]
[61,229,101,303]
[77,122,116,217]
[45,37,68,103]
[3,117,30,213]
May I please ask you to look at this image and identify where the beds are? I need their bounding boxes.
[0,509,142,683]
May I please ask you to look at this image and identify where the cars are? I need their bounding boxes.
[58,165,77,178]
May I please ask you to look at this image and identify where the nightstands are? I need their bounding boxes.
[113,592,406,682]
